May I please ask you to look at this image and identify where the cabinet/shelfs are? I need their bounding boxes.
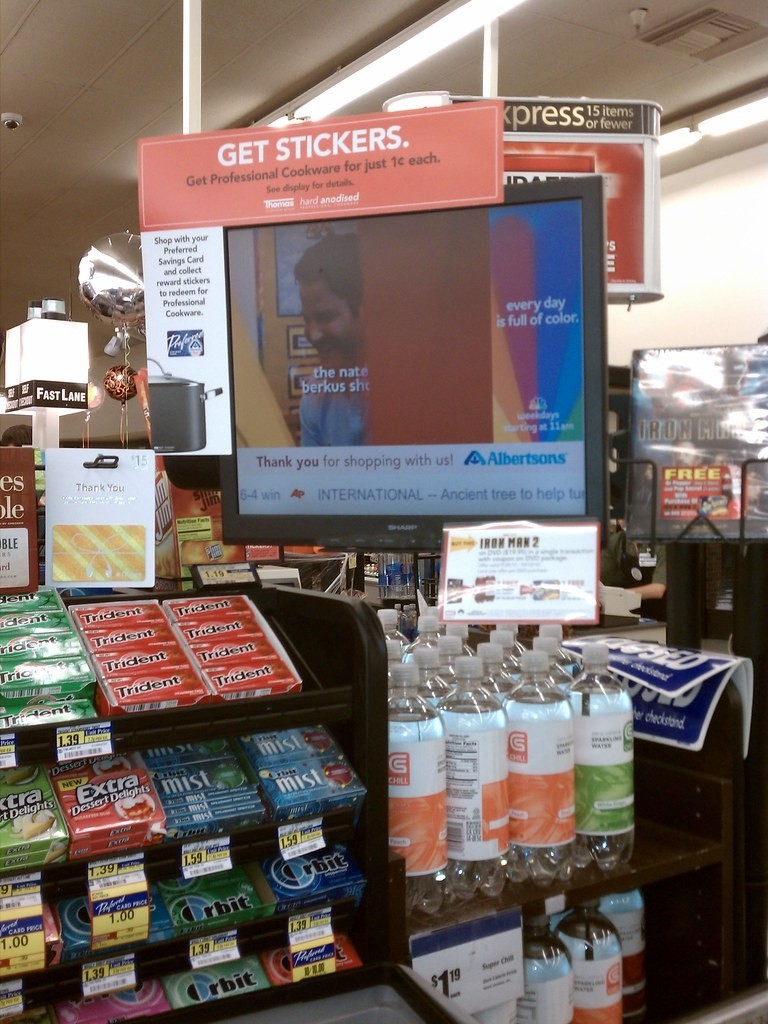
[0,575,768,1024]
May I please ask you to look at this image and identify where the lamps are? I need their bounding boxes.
[253,0,537,130]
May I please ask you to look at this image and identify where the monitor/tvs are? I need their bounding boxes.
[225,173,606,547]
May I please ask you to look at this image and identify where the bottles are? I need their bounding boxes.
[379,601,651,1024]
